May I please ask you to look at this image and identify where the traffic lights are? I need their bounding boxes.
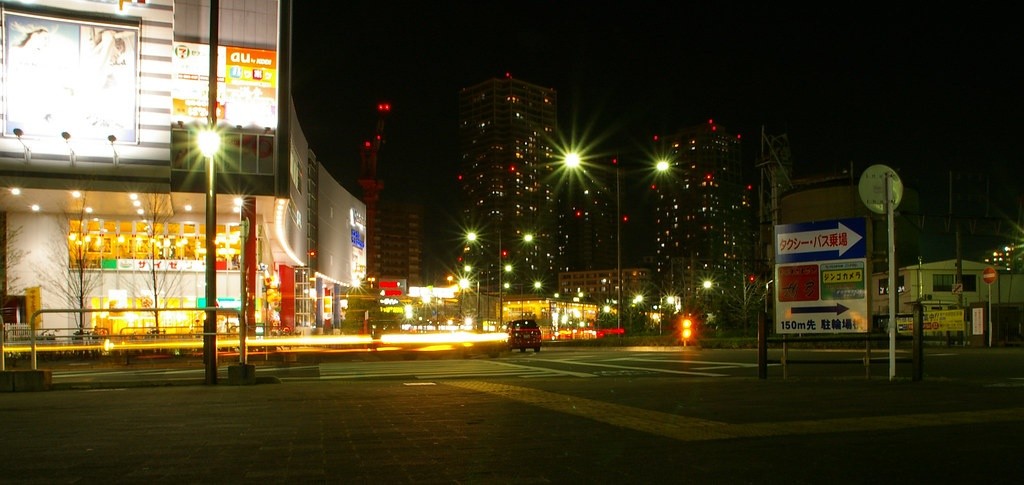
[681,318,692,341]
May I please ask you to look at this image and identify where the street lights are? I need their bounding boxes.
[654,156,698,312]
[196,126,220,387]
[466,231,483,333]
[562,152,624,339]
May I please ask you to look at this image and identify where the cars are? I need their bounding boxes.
[505,320,542,354]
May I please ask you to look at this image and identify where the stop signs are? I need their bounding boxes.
[982,265,997,286]
